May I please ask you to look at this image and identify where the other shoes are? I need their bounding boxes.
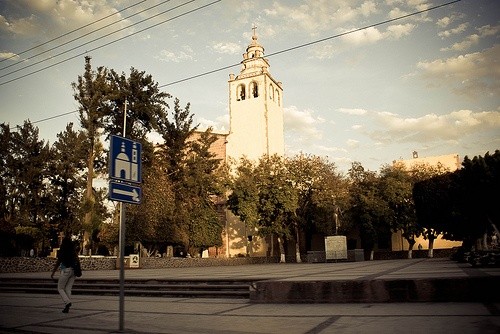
[62,303,71,313]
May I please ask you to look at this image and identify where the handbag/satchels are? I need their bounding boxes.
[72,250,82,277]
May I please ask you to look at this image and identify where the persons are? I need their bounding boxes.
[51,237,82,314]
